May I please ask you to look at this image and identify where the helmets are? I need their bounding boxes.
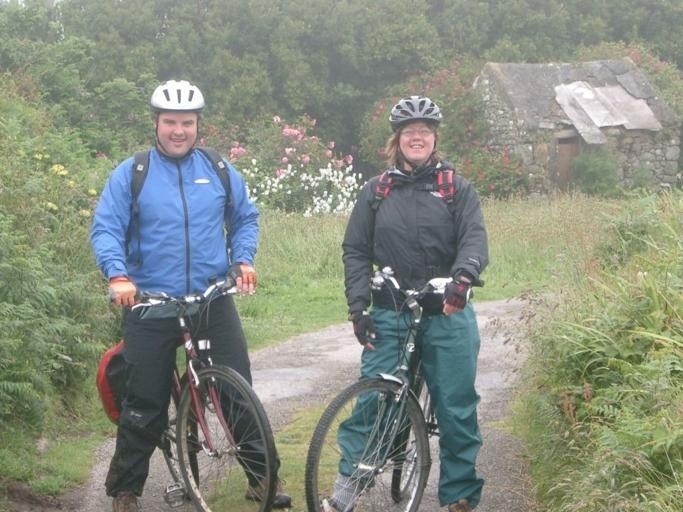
[389,95,443,123]
[151,80,205,111]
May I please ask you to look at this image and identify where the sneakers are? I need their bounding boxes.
[322,499,353,512]
[245,479,291,507]
[448,499,472,512]
[112,491,140,512]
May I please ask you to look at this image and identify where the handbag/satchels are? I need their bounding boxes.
[97,341,130,425]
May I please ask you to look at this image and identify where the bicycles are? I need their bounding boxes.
[305,266,484,512]
[109,276,278,512]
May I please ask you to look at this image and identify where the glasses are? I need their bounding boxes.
[403,126,431,138]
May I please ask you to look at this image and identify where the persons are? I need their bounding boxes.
[89,79,291,512]
[319,94,489,512]
